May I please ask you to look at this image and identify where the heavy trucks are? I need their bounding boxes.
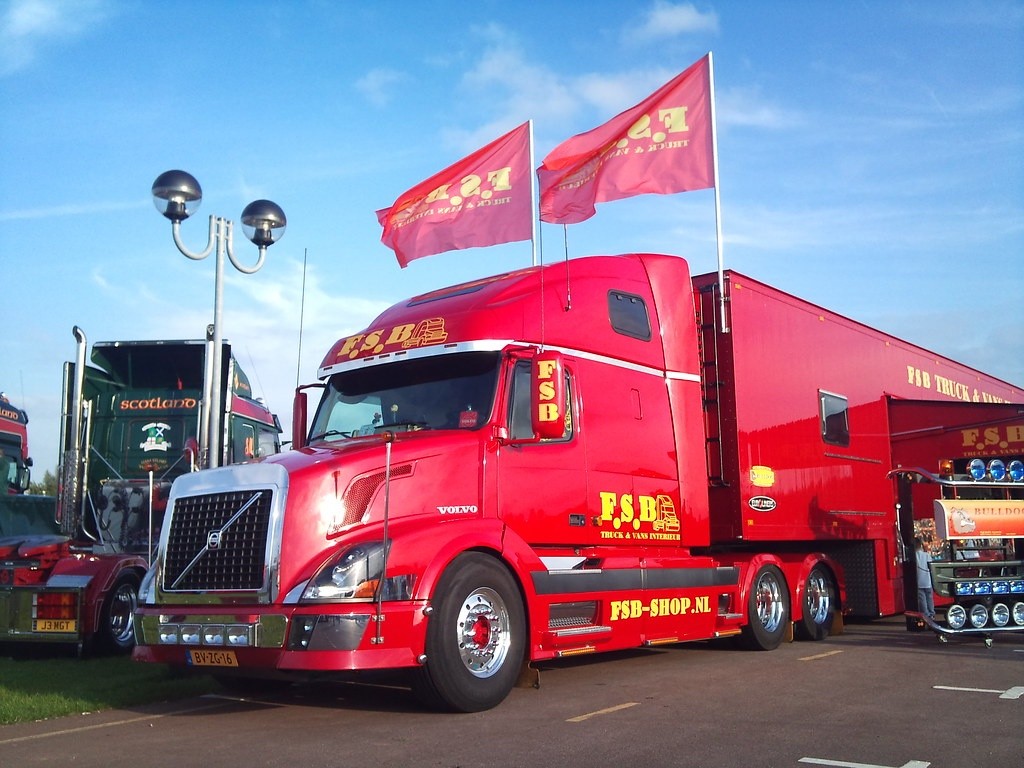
[0,324,295,657]
[130,248,1024,713]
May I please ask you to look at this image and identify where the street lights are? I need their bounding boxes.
[152,171,288,475]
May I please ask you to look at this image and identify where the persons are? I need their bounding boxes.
[913,538,937,618]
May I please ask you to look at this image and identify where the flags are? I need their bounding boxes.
[536,52,718,223]
[375,119,534,269]
